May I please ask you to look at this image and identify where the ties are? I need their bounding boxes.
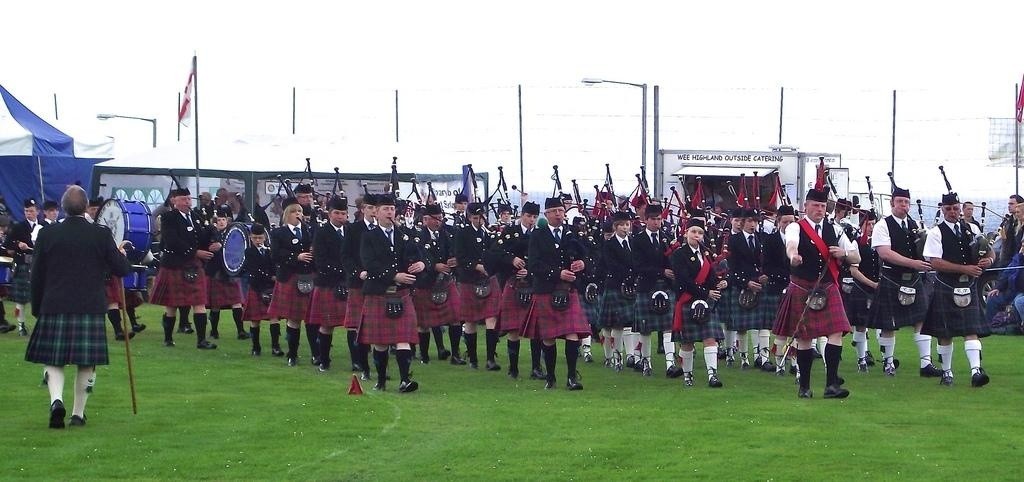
[293,227,302,242]
[337,229,342,240]
[954,224,961,237]
[651,233,658,247]
[815,225,820,235]
[902,220,907,232]
[369,224,376,229]
[554,228,561,243]
[748,235,755,250]
[386,229,393,242]
[623,240,630,253]
[186,213,192,226]
[260,248,266,256]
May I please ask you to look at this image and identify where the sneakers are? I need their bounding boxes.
[18,329,27,336]
[0,320,16,333]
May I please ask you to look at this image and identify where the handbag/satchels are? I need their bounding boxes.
[338,285,349,299]
[184,268,200,282]
[385,301,404,319]
[428,282,670,313]
[690,299,711,323]
[298,279,313,294]
[739,288,758,309]
[262,291,273,305]
[898,286,917,307]
[953,287,971,307]
[804,290,828,311]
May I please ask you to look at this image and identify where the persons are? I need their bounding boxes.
[556,186,1024,401]
[149,181,593,393]
[1,195,146,341]
[24,183,136,429]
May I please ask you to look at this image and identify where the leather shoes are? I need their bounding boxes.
[605,354,652,375]
[352,360,418,393]
[685,372,695,386]
[719,346,800,384]
[708,374,723,387]
[197,340,216,350]
[919,363,953,386]
[237,331,331,372]
[438,349,502,372]
[507,365,584,390]
[420,354,430,364]
[799,387,812,398]
[972,373,990,388]
[116,332,135,340]
[133,324,146,332]
[584,351,593,362]
[666,365,683,378]
[857,350,899,377]
[184,322,193,333]
[49,399,67,429]
[70,414,87,426]
[210,330,219,338]
[164,340,176,347]
[824,384,849,399]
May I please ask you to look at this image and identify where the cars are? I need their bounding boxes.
[978,233,1003,320]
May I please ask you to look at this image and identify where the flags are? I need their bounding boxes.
[1015,73,1024,123]
[177,72,193,128]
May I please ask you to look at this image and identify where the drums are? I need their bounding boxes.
[222,223,270,276]
[93,197,153,264]
[123,265,147,292]
[1,255,14,286]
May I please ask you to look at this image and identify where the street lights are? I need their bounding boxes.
[95,109,158,149]
[578,74,649,191]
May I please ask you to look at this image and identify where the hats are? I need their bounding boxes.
[686,219,705,230]
[859,210,876,223]
[42,200,59,210]
[251,223,264,235]
[943,192,959,205]
[498,192,572,215]
[892,188,911,199]
[614,212,633,221]
[729,205,795,217]
[217,210,228,219]
[806,189,827,202]
[89,200,101,207]
[645,205,664,214]
[174,188,190,196]
[282,184,483,215]
[25,198,37,208]
